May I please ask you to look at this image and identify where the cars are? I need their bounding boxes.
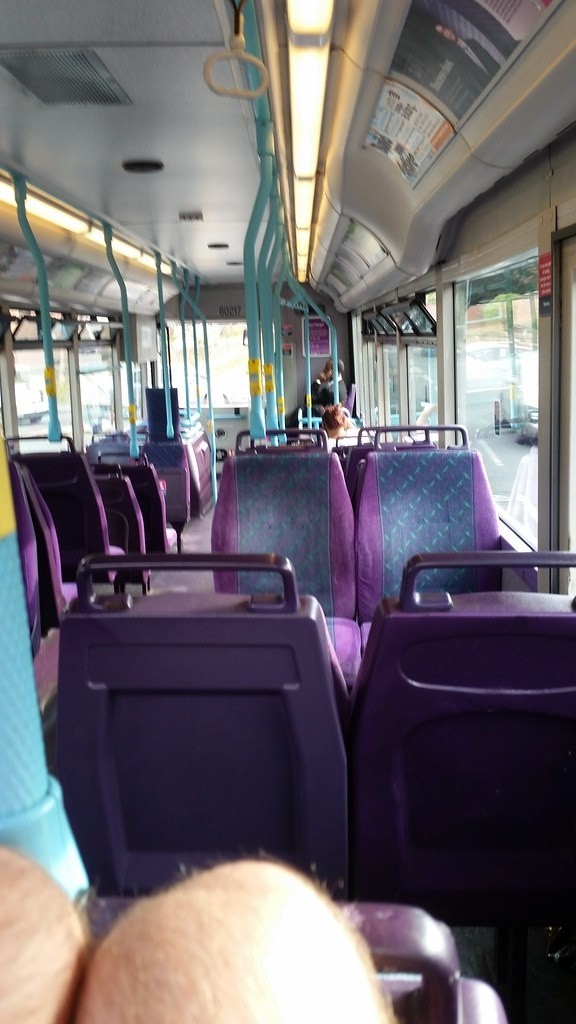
[466,341,533,403]
[498,351,539,445]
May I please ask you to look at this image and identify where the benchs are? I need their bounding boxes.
[2,425,576,1024]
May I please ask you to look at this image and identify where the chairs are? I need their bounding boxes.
[297,384,356,430]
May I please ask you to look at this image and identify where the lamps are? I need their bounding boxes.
[273,0,349,282]
[1,174,171,275]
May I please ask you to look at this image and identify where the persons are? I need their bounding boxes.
[304,359,348,417]
[0,846,395,1024]
[320,406,365,451]
